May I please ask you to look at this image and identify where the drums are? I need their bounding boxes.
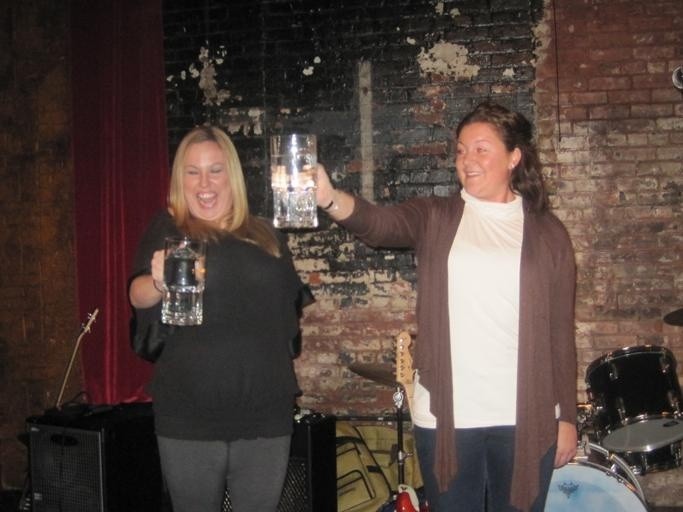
[587,344,683,453]
[541,459,647,512]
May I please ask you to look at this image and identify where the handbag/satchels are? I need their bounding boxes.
[332,420,393,512]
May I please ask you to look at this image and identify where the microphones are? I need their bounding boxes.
[55,308,101,407]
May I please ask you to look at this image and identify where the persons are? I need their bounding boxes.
[297,101,579,512]
[126,123,315,512]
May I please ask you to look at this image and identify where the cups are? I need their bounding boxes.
[270,130,323,231]
[163,235,207,327]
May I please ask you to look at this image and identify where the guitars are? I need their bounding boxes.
[395,330,420,422]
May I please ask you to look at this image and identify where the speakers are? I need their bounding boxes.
[221,413,337,512]
[24,399,161,512]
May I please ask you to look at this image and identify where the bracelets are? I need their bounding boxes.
[150,279,165,296]
[321,185,340,211]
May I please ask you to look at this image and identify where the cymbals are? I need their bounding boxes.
[344,359,403,389]
